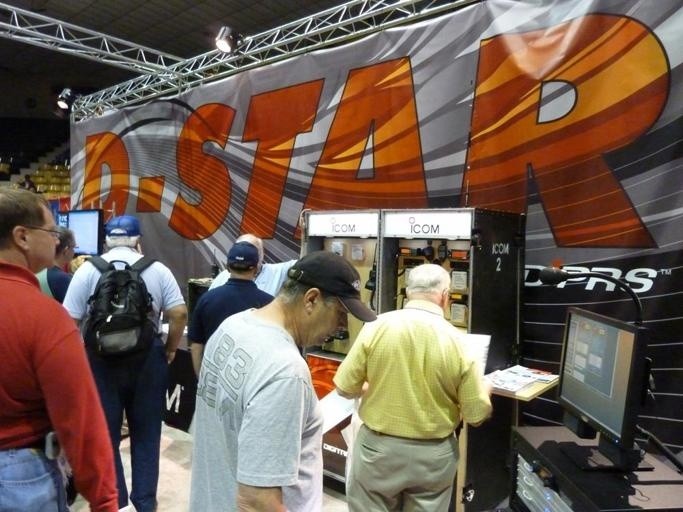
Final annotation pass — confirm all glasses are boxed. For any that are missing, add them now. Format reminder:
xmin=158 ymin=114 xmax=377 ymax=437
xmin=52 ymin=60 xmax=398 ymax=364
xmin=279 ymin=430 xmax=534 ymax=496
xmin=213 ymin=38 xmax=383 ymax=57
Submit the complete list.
xmin=22 ymin=225 xmax=64 ymax=240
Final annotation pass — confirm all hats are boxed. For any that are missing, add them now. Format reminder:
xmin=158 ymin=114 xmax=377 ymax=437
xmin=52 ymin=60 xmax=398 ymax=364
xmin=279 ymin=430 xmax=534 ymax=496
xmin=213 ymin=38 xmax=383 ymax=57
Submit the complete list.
xmin=287 ymin=250 xmax=377 ymax=321
xmin=104 ymin=216 xmax=141 ymax=237
xmin=228 ymin=242 xmax=258 ymax=270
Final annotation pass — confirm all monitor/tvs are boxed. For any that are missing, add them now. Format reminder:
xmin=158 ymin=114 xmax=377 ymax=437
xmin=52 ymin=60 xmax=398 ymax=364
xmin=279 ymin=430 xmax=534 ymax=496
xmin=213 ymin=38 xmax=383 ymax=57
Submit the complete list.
xmin=555 ymin=304 xmax=656 ymax=471
xmin=66 ymin=209 xmax=103 ymax=256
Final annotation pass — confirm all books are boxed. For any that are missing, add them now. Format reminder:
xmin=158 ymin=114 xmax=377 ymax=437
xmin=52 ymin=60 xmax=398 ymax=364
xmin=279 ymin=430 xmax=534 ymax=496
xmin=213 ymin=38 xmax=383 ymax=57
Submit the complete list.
xmin=485 ymin=363 xmax=559 ymax=402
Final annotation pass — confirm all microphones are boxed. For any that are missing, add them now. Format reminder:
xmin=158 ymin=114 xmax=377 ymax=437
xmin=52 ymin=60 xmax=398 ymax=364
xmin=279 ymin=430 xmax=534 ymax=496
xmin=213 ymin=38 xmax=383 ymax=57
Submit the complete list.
xmin=539 ymin=267 xmax=656 ymax=403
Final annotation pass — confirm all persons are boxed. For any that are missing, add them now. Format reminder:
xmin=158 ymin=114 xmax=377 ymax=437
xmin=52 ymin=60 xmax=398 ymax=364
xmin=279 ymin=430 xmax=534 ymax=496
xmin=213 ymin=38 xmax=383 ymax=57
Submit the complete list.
xmin=187 ymin=241 xmax=276 ymax=382
xmin=185 ymin=251 xmax=378 ymax=512
xmin=1 ymin=184 xmax=118 ymax=511
xmin=36 ymin=225 xmax=76 ymax=306
xmin=331 ymin=263 xmax=493 ymax=512
xmin=60 ymin=214 xmax=189 ymax=512
xmin=206 ymin=208 xmax=311 ymax=298
xmin=20 ymin=175 xmax=36 ymax=192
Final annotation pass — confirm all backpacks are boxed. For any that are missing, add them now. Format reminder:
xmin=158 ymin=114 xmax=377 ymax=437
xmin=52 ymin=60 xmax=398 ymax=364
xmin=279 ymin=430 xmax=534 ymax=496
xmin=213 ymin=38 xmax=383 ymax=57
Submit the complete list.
xmin=79 ymin=255 xmax=157 ymax=358
